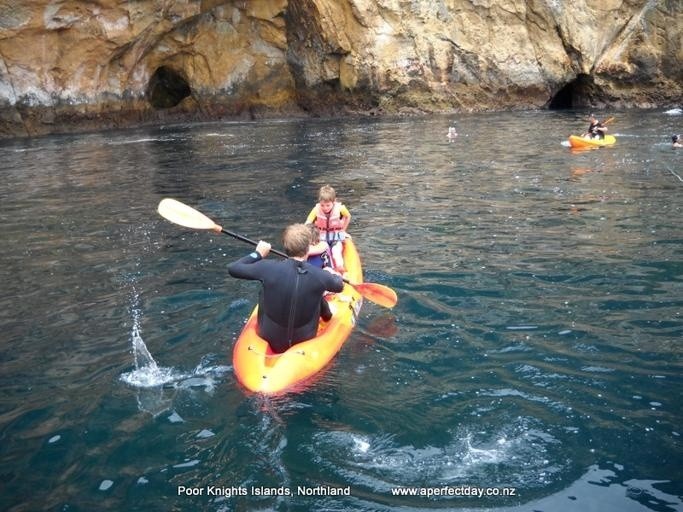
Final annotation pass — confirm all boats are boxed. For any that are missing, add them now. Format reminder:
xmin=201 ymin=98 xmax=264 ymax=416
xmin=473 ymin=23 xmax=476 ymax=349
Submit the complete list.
xmin=231 ymin=229 xmax=363 ymax=396
xmin=567 ymin=134 xmax=617 ymax=148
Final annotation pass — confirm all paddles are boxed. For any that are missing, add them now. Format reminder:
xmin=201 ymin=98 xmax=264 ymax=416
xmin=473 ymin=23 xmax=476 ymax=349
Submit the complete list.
xmin=581 ymin=116 xmax=614 ymax=136
xmin=157 ymin=198 xmax=398 ymax=308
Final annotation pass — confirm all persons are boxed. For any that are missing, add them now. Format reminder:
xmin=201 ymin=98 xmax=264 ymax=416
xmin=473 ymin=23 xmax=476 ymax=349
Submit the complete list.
xmin=226 ymin=223 xmax=343 ymax=353
xmin=671 ymin=134 xmax=683 ymax=148
xmin=304 ymin=185 xmax=350 ymax=272
xmin=585 ymin=113 xmax=607 ymax=140
